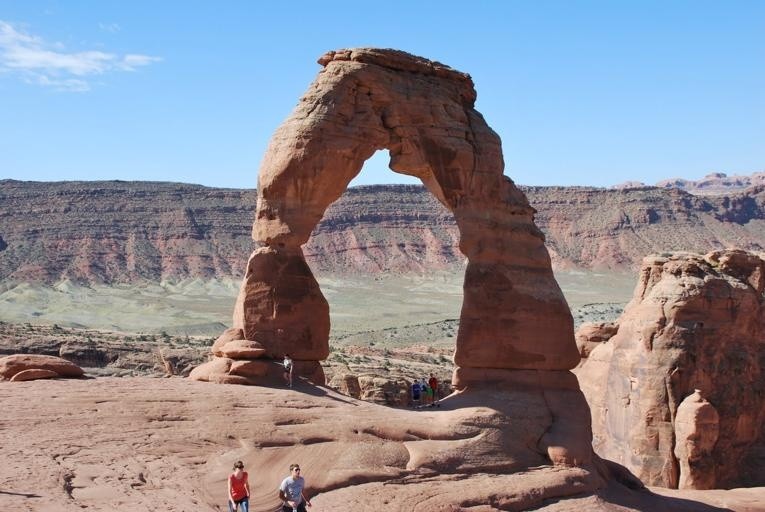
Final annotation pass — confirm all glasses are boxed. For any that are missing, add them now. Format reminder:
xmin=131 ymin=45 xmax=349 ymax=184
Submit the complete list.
xmin=236 ymin=466 xmax=244 ymax=469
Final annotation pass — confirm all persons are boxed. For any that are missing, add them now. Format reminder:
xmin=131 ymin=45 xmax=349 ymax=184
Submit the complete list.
xmin=420 ymin=378 xmax=428 ymax=405
xmin=282 ymin=353 xmax=294 ymax=387
xmin=279 ymin=464 xmax=313 ymax=512
xmin=426 ymin=383 xmax=432 ymax=404
xmin=411 ymin=379 xmax=420 ymax=409
xmin=429 ymin=373 xmax=440 ymax=407
xmin=228 ymin=460 xmax=250 ymax=511
